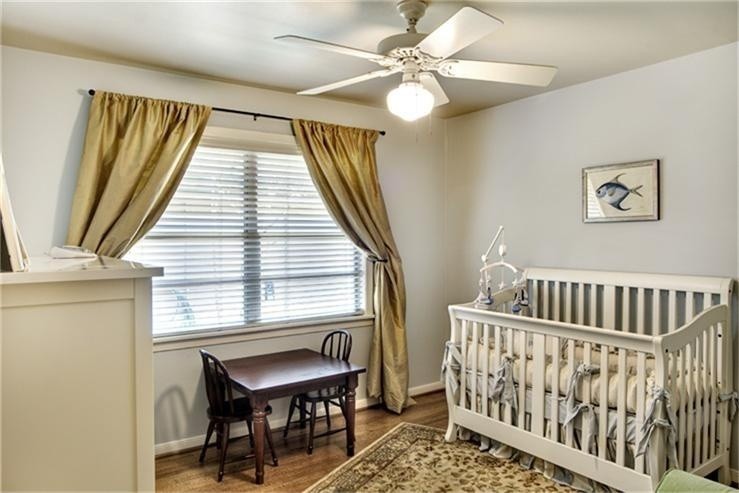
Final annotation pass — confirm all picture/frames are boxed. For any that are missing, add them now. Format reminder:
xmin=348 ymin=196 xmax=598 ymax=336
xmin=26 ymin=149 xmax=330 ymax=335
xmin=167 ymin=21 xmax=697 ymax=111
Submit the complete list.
xmin=582 ymin=157 xmax=661 ymax=225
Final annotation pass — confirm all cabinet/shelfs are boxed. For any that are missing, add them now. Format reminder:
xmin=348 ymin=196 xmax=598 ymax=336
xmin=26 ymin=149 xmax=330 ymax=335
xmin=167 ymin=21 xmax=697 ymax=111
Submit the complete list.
xmin=1 ymin=266 xmax=164 ymax=493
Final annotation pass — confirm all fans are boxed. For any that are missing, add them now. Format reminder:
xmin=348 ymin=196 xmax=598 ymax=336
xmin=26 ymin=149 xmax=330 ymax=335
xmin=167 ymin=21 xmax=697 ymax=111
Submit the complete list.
xmin=275 ymin=0 xmax=559 ymax=98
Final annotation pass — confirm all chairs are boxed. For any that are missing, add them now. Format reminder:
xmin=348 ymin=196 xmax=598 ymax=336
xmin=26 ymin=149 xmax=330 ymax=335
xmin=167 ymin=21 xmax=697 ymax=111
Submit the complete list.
xmin=284 ymin=330 xmax=353 ymax=454
xmin=199 ymin=348 xmax=278 ymax=481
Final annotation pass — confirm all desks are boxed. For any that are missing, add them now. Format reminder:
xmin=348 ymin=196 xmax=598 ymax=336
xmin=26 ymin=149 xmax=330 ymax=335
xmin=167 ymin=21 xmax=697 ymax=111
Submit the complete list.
xmin=206 ymin=348 xmax=367 ymax=484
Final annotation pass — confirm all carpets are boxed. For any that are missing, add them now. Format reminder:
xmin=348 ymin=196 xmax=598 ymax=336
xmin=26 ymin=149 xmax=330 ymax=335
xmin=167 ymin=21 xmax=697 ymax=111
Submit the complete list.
xmin=303 ymin=423 xmax=589 ymax=493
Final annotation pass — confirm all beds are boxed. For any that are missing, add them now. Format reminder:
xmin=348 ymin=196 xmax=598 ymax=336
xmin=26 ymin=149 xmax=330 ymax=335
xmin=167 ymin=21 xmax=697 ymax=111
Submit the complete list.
xmin=443 ymin=265 xmax=736 ymax=493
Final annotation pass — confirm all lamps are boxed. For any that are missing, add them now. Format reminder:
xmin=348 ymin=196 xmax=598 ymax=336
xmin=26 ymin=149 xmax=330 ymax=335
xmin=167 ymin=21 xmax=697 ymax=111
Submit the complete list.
xmin=385 ymin=63 xmax=434 ymax=122
